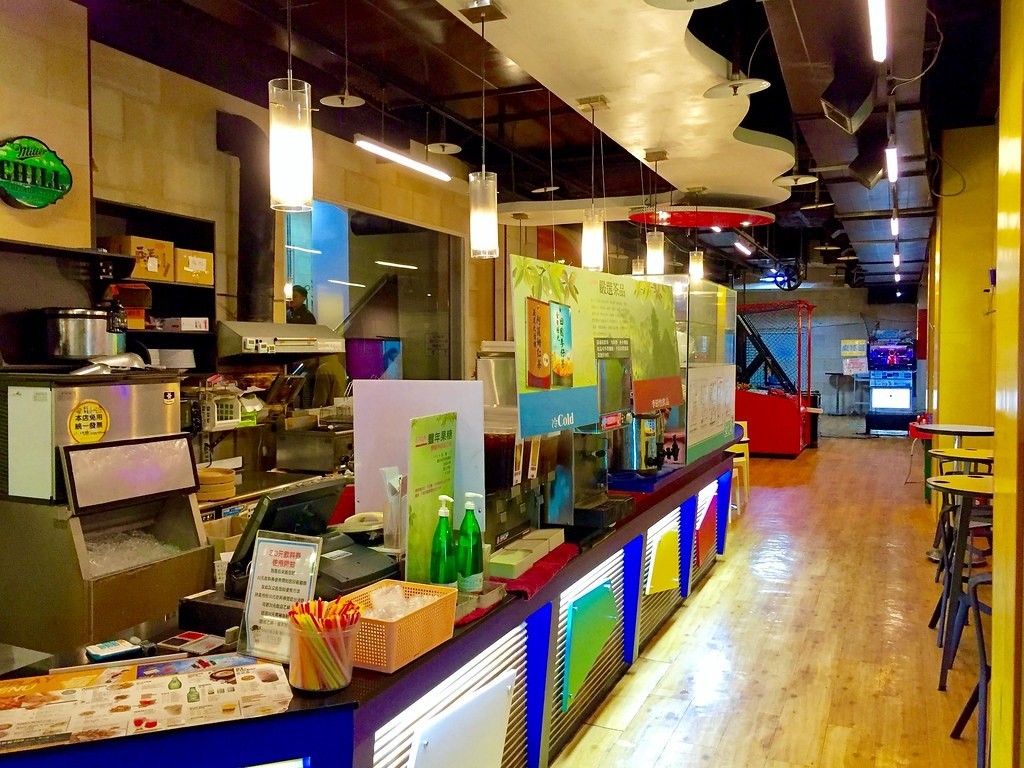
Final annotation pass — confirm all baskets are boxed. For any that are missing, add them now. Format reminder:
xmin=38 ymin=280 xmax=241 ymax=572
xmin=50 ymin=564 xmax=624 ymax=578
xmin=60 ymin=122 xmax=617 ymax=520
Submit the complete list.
xmin=325 ymin=578 xmax=459 ymax=675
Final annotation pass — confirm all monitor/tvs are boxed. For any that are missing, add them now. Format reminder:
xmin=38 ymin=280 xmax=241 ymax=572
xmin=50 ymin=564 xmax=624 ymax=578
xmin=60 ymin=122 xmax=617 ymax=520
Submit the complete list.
xmin=867 ymin=339 xmax=917 ymax=371
xmin=229 ymin=477 xmax=347 ymax=568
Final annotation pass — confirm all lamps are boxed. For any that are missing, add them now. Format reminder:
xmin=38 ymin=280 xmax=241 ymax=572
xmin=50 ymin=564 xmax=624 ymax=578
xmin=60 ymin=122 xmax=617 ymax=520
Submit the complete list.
xmin=686 ymin=187 xmax=707 ymax=280
xmin=269 ymin=0 xmax=314 ymax=211
xmin=989 ymin=268 xmax=996 ymax=286
xmin=459 ymin=0 xmax=507 ymax=258
xmin=645 ymin=150 xmax=665 ymax=274
xmin=577 ymin=94 xmax=609 ymax=271
xmin=353 ymin=84 xmax=452 ymax=181
xmin=860 ymin=0 xmax=903 ymax=281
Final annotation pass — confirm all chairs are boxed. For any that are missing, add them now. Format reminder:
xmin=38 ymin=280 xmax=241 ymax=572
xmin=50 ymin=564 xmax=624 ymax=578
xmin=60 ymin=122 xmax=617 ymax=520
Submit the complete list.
xmin=927 ymin=470 xmax=996 ymax=768
xmin=725 ymin=420 xmax=750 ymax=504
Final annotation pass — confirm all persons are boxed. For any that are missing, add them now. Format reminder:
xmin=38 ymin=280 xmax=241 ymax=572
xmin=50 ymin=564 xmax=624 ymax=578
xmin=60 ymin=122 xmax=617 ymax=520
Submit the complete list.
xmin=286 ymin=285 xmax=316 ymax=325
xmin=385 ymin=348 xmax=398 ymax=379
xmin=312 ymin=355 xmax=347 ymax=407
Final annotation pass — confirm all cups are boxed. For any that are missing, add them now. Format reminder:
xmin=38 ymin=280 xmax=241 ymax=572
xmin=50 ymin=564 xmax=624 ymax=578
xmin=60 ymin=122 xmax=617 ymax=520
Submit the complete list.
xmin=287 ymin=619 xmax=362 ymax=692
xmin=222 ymin=704 xmax=236 ymax=716
xmin=165 ymin=704 xmax=183 ymax=716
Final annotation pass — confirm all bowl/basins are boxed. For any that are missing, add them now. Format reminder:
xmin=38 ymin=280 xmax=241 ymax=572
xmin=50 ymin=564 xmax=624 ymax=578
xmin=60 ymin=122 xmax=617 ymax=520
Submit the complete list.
xmin=140 ymin=698 xmax=155 ymax=706
xmin=44 ymin=302 xmax=127 ymax=365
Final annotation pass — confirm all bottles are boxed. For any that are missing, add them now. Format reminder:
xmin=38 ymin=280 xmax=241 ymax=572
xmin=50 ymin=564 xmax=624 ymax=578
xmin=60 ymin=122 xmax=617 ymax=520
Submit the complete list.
xmin=168 ymin=677 xmax=182 ymax=689
xmin=186 ymin=687 xmax=200 ymax=704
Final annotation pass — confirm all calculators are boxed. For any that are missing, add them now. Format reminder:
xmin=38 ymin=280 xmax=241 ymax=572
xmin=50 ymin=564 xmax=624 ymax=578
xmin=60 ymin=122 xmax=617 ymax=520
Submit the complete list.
xmin=85 ymin=639 xmax=141 ymax=663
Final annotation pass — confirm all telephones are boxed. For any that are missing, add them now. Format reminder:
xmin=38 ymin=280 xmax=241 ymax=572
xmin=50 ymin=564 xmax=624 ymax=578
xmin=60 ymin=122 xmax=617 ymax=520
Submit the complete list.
xmin=326 ymin=511 xmax=384 ymax=547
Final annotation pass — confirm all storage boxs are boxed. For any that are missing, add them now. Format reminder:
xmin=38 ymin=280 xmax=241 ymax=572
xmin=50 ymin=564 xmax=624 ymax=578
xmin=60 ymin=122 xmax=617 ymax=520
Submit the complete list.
xmin=202 ymin=515 xmax=251 ymax=563
xmin=97 ymin=234 xmax=214 ymax=330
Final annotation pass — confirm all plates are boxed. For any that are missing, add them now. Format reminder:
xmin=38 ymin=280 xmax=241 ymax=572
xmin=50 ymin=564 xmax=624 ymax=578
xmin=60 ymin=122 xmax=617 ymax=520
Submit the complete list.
xmin=196 ymin=468 xmax=237 ymax=501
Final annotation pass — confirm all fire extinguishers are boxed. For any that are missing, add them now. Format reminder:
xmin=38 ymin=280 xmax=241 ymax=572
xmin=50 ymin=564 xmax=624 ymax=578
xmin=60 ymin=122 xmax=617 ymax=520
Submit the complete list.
xmin=970 ymin=496 xmax=987 ymax=536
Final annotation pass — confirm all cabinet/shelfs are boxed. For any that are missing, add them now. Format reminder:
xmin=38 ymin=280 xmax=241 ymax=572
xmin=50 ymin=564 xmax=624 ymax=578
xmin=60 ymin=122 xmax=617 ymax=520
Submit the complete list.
xmin=100 ymin=275 xmax=215 ymax=335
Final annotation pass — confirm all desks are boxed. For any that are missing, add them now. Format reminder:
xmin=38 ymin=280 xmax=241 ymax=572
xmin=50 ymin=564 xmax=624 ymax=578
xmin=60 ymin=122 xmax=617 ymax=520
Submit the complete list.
xmin=916 ymin=425 xmax=994 ymax=689
xmin=905 ymin=422 xmax=933 ymax=484
xmin=825 ymin=372 xmax=847 ymax=416
